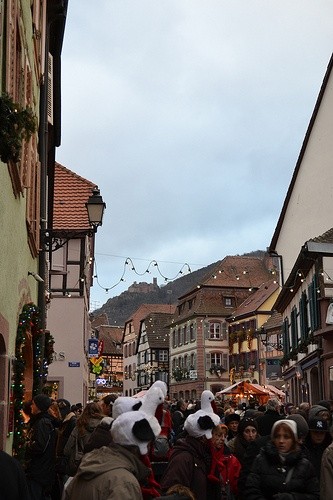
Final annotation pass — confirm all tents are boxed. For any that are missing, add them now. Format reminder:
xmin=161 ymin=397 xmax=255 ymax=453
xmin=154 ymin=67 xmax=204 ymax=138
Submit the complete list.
xmin=262 ymin=385 xmax=285 ymax=403
xmin=215 ymin=381 xmax=268 ymax=406
xmin=250 ymin=383 xmax=275 ymax=398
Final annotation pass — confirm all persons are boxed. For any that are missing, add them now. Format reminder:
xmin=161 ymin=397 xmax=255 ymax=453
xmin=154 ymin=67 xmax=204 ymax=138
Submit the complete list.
xmin=0 ymin=380 xmax=333 ymax=500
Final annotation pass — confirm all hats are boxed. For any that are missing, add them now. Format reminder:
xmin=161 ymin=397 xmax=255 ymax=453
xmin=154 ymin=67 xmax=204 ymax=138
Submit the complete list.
xmin=286 ymin=414 xmax=307 ymax=435
xmin=183 ymin=390 xmax=220 ymax=439
xmin=110 ymin=388 xmax=165 ymax=455
xmin=215 ymin=420 xmax=228 ymax=431
xmin=271 ymin=419 xmax=298 ymax=444
xmin=33 ymin=395 xmax=51 ymax=412
xmin=224 ymin=414 xmax=240 ymax=424
xmin=112 ymin=380 xmax=167 ymax=421
xmin=238 ymin=418 xmax=257 ymax=434
xmin=309 ymin=416 xmax=328 ymax=432
